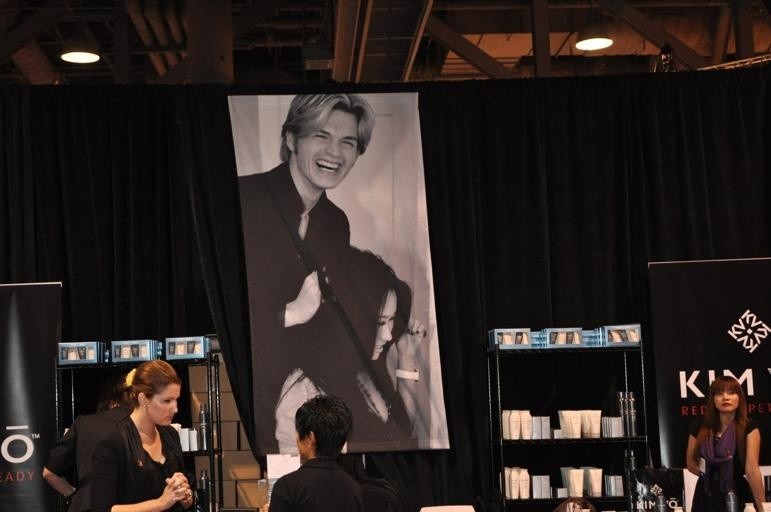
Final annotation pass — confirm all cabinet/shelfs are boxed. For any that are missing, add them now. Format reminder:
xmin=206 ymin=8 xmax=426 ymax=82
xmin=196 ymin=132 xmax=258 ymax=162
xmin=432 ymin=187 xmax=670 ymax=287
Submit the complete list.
xmin=488 ymin=340 xmax=652 ymax=511
xmin=50 ymin=352 xmax=224 ymax=510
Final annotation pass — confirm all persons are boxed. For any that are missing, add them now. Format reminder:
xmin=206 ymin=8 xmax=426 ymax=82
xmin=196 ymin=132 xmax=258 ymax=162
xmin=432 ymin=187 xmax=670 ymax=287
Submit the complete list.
xmin=41 ymin=369 xmax=136 ymax=511
xmin=237 ymin=92 xmax=376 ymax=454
xmin=71 ymin=361 xmax=197 ymax=512
xmin=686 ymin=376 xmax=766 ymax=512
xmin=263 ymin=394 xmax=365 ymax=512
xmin=268 ymin=246 xmax=428 ymax=453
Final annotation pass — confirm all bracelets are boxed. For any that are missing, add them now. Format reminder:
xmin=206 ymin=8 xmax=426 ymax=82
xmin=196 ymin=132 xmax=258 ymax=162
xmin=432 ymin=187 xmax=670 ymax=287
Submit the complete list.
xmin=394 ymin=368 xmax=420 ymax=383
xmin=64 ymin=487 xmax=76 ymax=499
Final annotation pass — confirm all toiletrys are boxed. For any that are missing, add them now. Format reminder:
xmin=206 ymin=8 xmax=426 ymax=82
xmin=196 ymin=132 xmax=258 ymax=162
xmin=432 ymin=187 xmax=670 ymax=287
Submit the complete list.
xmin=499 ymin=466 xmax=530 ymax=499
xmin=560 ymin=466 xmax=603 ymax=498
xmin=558 ymin=409 xmax=601 ymax=439
xmin=502 ymin=410 xmax=532 ymax=440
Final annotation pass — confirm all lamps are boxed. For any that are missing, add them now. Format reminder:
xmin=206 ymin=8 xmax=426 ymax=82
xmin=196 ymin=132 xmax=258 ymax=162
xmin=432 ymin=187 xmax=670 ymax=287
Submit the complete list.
xmin=60 ymin=27 xmax=102 ymax=65
xmin=573 ymin=2 xmax=616 ymax=52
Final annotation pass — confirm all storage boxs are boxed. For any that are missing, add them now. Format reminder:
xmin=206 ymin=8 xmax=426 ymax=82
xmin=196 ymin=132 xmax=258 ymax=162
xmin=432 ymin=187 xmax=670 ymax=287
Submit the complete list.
xmin=188 ymin=363 xmax=268 ymax=511
